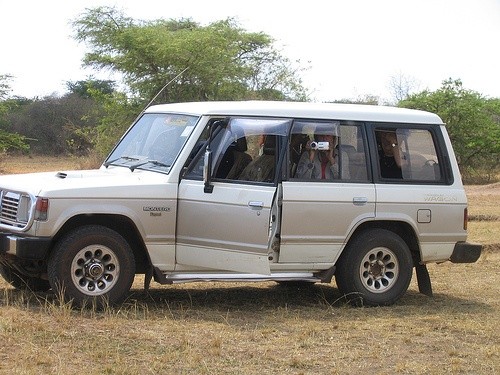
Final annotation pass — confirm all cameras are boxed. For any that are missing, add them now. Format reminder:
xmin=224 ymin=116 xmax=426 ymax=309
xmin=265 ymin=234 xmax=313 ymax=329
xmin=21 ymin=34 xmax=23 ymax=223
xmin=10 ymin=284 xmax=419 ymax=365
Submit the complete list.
xmin=310 ymin=141 xmax=329 ymax=151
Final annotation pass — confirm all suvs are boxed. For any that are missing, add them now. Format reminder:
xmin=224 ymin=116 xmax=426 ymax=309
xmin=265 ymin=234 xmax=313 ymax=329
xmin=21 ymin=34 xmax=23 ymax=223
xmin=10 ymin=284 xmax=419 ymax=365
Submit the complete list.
xmin=0 ymin=98 xmax=484 ymax=311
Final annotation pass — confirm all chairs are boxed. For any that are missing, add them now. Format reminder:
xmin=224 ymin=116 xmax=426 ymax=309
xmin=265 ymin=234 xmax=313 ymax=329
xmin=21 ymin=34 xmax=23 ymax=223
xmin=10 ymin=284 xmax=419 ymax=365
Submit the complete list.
xmin=334 ymin=143 xmax=365 ymax=181
xmin=238 ymin=125 xmax=278 ymax=181
xmin=399 ymin=152 xmax=434 ymax=182
xmin=287 ymin=129 xmax=312 ymax=178
xmin=192 ymin=123 xmax=253 ymax=182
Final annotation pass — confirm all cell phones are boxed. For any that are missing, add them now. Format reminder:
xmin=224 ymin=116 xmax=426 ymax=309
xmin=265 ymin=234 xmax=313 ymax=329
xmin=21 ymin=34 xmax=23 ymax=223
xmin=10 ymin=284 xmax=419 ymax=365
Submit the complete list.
xmin=392 ymin=144 xmax=404 ymax=166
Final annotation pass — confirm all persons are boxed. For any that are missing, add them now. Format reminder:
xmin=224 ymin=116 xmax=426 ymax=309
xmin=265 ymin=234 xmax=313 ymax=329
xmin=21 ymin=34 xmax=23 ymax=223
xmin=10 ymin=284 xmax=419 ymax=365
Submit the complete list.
xmin=293 ymin=124 xmax=352 ymax=181
xmin=354 ymin=124 xmax=414 ymax=182
xmin=254 ymin=122 xmax=275 ymax=153
